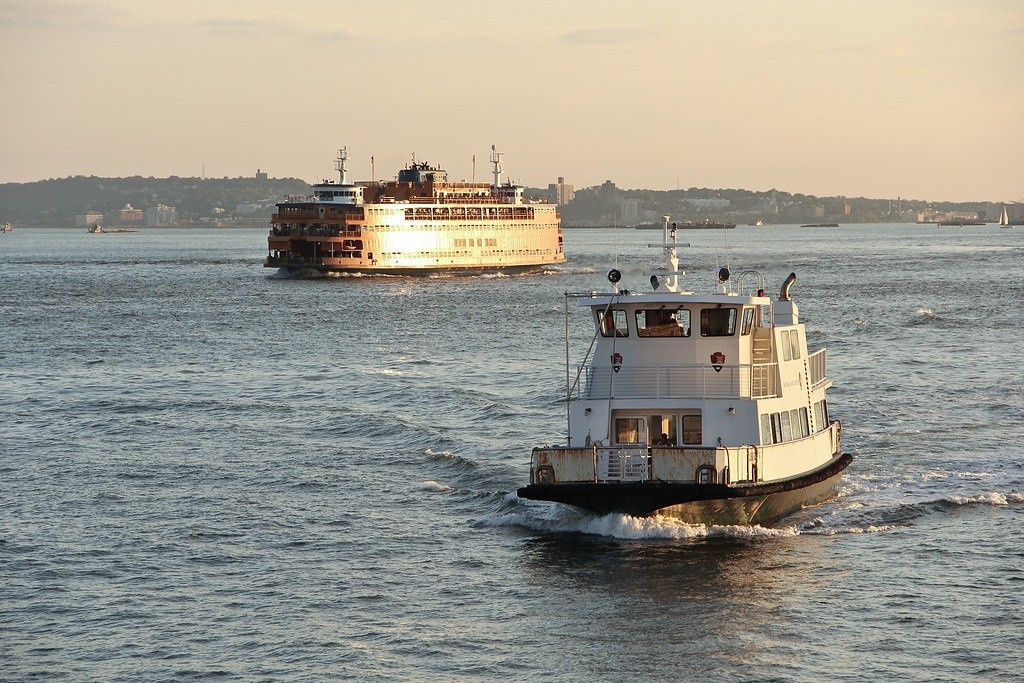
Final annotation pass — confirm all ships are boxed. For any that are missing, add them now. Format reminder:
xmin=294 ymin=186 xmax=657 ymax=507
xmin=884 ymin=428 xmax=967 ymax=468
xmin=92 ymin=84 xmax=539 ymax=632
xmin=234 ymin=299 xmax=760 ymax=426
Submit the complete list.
xmin=263 ymin=144 xmax=569 ymax=276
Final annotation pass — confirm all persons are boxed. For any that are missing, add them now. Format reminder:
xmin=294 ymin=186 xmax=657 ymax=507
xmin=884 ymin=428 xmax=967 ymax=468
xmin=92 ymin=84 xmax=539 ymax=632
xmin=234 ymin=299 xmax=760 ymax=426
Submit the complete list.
xmin=659 ymin=311 xmax=677 ymax=325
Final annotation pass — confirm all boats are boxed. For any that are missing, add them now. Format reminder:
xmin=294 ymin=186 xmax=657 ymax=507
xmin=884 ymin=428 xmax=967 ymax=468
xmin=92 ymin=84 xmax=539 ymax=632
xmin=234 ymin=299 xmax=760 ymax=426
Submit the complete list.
xmin=87 ymin=223 xmax=104 ymax=233
xmin=0 ymin=222 xmax=13 ymax=232
xmin=514 ymin=213 xmax=856 ymax=531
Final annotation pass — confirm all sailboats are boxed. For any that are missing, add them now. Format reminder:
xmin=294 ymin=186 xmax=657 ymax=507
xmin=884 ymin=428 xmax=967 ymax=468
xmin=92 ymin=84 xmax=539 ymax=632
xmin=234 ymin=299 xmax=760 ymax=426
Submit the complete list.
xmin=999 ymin=207 xmax=1015 ymax=229
